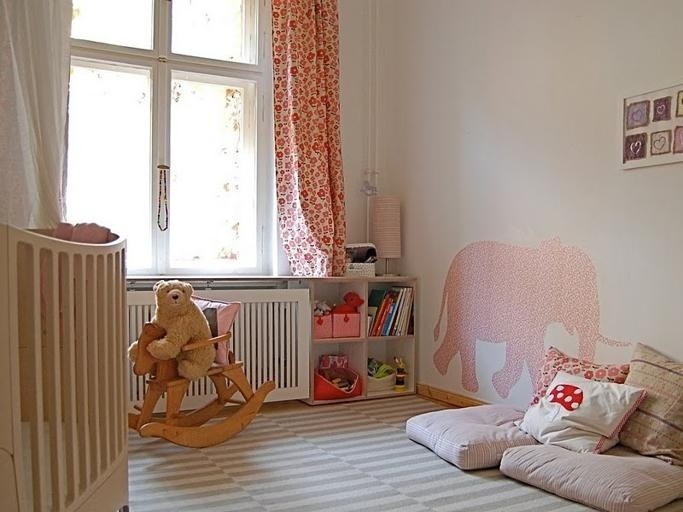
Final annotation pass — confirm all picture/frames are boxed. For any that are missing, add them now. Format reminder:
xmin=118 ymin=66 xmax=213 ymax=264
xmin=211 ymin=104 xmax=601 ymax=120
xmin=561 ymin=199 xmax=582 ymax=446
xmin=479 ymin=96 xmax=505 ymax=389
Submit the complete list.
xmin=618 ymin=77 xmax=683 ymax=171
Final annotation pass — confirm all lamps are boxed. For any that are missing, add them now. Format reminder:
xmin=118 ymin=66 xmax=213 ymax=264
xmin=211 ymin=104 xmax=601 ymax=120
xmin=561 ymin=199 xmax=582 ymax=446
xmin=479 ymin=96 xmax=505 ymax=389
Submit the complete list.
xmin=371 ymin=197 xmax=402 ymax=276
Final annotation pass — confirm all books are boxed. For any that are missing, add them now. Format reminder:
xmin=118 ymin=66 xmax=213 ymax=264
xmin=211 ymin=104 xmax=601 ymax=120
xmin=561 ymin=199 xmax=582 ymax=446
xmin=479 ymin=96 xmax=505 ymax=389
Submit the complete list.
xmin=368 ymin=285 xmax=413 ymax=335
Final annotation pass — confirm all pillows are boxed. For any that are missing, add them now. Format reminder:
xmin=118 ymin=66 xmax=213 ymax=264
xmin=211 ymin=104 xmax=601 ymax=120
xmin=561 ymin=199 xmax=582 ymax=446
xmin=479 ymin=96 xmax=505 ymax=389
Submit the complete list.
xmin=512 ymin=342 xmax=683 ymax=467
xmin=191 ymin=295 xmax=242 ymax=368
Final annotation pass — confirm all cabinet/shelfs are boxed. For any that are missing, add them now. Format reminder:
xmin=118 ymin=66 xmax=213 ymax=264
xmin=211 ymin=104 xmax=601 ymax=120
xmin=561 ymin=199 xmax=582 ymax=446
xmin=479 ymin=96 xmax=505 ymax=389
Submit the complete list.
xmin=287 ymin=279 xmax=417 ymax=407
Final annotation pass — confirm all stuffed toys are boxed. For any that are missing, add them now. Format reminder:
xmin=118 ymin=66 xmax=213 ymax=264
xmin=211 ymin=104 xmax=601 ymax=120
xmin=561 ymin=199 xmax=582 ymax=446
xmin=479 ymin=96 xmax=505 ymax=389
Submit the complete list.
xmin=128 ymin=278 xmax=216 ymax=380
